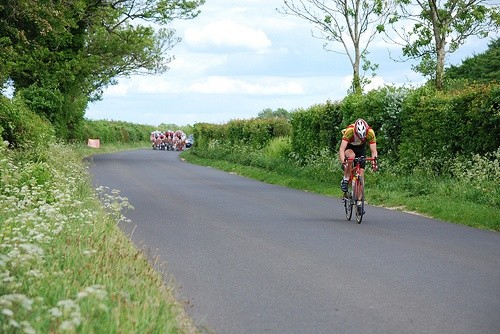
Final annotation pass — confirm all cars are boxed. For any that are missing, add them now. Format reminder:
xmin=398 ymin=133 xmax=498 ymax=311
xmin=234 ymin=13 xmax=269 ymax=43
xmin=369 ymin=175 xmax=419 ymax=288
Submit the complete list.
xmin=186 ymin=135 xmax=194 ymax=147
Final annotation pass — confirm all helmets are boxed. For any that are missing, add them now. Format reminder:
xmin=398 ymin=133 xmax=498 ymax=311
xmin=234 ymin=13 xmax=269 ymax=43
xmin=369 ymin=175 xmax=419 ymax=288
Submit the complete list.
xmin=354 ymin=118 xmax=369 ymax=142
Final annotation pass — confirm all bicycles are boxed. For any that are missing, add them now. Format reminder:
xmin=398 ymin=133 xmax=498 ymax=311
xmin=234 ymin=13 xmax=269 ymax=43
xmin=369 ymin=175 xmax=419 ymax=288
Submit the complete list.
xmin=343 ymin=156 xmax=378 ymax=223
xmin=152 ymin=138 xmax=183 ymax=151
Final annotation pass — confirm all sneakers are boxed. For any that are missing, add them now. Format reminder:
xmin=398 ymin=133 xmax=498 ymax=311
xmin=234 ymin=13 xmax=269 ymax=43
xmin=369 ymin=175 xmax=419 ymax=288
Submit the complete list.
xmin=341 ymin=180 xmax=349 ymax=192
xmin=356 ymin=205 xmax=365 ymax=215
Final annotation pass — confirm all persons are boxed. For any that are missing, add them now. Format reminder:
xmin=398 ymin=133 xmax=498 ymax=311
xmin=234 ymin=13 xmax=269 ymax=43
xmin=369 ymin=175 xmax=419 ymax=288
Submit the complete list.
xmin=149 ymin=129 xmax=186 ymax=151
xmin=339 ymin=117 xmax=377 ymax=215
